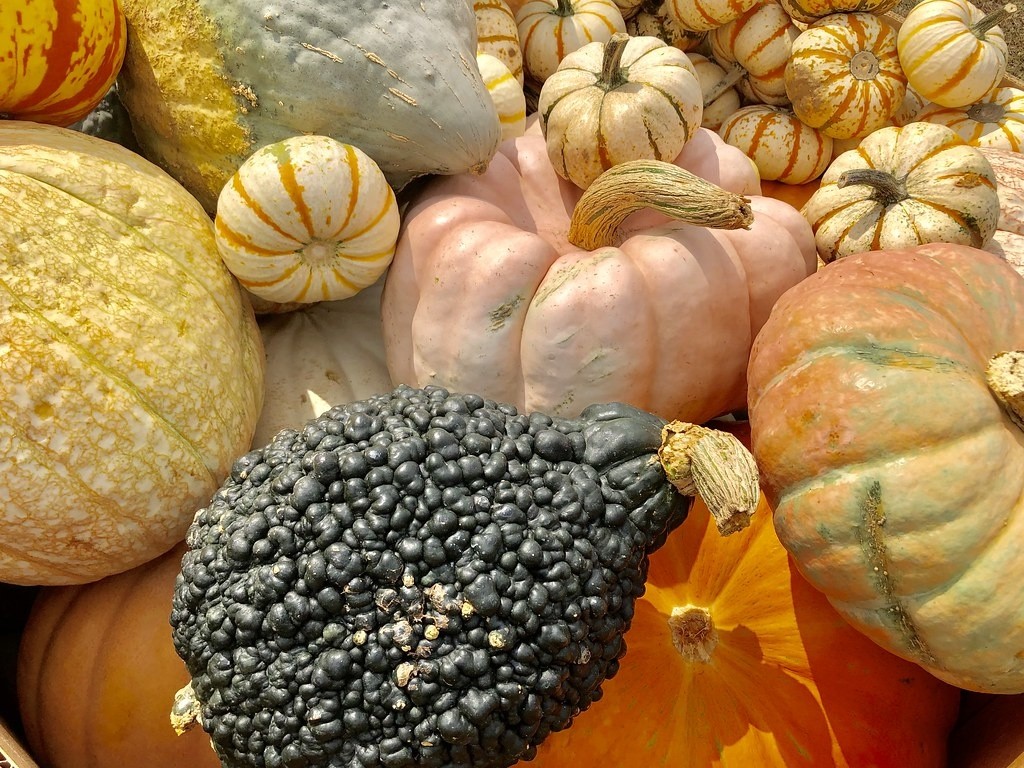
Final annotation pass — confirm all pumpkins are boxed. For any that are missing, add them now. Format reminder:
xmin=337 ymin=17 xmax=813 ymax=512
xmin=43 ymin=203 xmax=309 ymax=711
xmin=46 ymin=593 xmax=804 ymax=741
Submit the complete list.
xmin=0 ymin=0 xmax=1024 ymax=768
